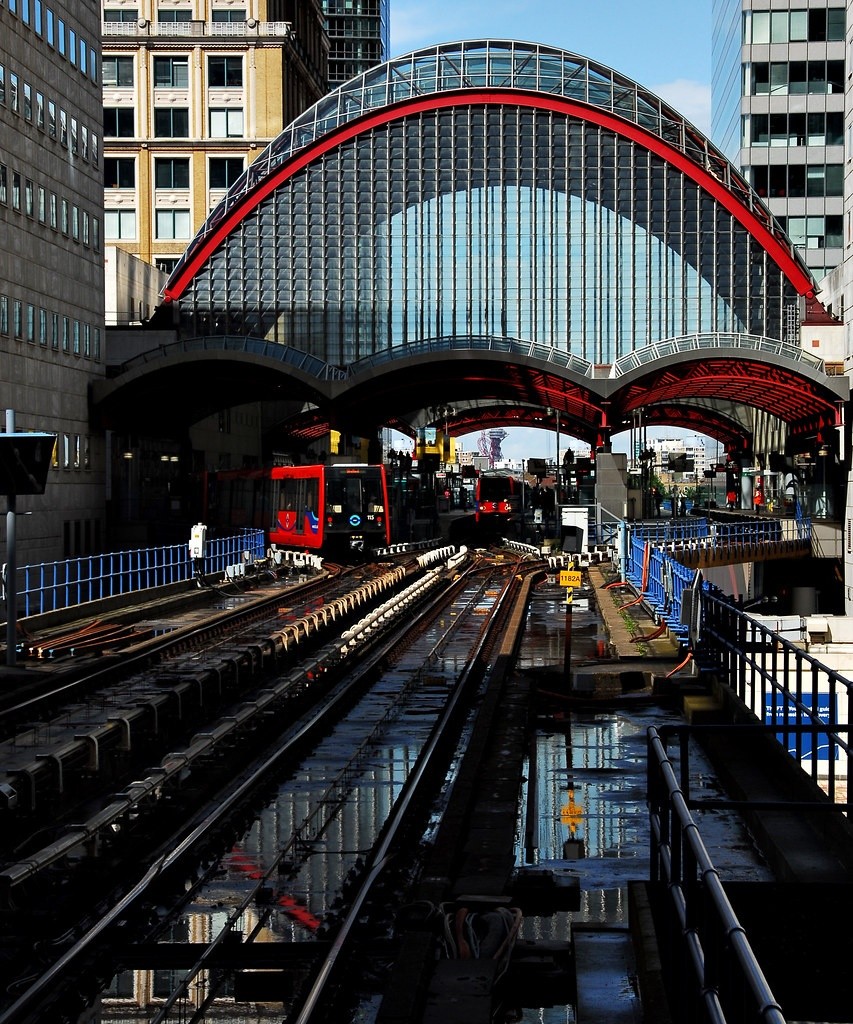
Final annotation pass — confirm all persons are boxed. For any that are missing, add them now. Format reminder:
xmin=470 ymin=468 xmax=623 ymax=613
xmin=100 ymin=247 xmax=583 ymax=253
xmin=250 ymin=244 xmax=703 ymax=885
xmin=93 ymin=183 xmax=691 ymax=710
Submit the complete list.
xmin=444 ymin=488 xmax=451 ymax=514
xmin=753 ymin=487 xmax=764 ymax=516
xmin=530 ymin=485 xmax=554 ymax=523
xmin=461 ymin=487 xmax=469 ymax=511
xmin=653 ymin=486 xmax=664 ymax=519
xmin=728 ymin=490 xmax=736 ymax=511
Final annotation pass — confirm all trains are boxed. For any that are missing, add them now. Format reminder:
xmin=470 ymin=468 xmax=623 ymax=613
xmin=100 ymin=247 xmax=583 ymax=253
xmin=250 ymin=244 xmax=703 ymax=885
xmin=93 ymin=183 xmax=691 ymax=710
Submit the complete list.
xmin=207 ymin=464 xmax=389 ymax=566
xmin=474 ymin=475 xmax=528 ymax=524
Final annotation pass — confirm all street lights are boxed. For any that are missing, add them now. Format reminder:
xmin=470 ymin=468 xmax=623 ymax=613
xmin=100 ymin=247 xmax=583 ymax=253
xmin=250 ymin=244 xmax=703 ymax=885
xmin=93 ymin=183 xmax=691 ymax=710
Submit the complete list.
xmin=697 ymin=484 xmax=700 ymax=504
xmin=0 ymin=410 xmax=57 ymax=667
xmin=546 ymin=407 xmax=560 ymax=521
